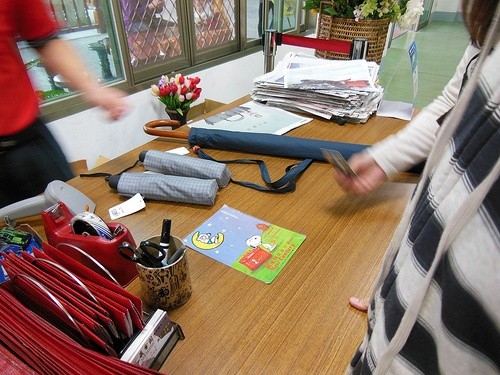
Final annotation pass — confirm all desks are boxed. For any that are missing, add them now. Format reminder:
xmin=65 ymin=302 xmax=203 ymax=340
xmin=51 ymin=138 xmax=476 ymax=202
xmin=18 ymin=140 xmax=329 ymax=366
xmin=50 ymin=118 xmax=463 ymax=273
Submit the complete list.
xmin=0 ymin=91 xmax=419 ymax=375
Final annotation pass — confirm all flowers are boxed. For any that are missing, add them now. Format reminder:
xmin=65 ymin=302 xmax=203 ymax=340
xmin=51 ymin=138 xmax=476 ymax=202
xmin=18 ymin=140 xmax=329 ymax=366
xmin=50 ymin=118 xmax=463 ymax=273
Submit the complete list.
xmin=300 ymin=0 xmax=425 ymax=35
xmin=149 ymin=70 xmax=203 ymax=117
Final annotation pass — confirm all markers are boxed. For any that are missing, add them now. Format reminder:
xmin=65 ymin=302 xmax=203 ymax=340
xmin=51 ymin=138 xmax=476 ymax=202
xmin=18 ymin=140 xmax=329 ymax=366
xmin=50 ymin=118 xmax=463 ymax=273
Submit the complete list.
xmin=158 ymin=219 xmax=171 ymax=266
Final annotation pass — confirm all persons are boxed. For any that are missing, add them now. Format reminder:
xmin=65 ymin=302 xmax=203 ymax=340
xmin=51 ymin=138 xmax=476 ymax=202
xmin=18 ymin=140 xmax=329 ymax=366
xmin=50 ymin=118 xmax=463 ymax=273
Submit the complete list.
xmin=333 ymin=0 xmax=500 ymax=375
xmin=193 ymin=0 xmax=218 ymax=33
xmin=120 ymin=0 xmax=175 ymax=33
xmin=0 ymin=0 xmax=126 ymax=210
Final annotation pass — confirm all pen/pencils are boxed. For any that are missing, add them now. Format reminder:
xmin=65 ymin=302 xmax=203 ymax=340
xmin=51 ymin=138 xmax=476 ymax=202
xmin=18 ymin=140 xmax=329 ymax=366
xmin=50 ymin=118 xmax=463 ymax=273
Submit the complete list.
xmin=168 ymin=245 xmax=185 ymax=265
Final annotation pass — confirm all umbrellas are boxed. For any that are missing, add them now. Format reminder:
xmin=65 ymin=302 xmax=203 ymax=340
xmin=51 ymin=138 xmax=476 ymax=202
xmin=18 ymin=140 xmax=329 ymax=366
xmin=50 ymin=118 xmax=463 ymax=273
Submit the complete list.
xmin=144 ymin=118 xmax=426 ymax=173
xmin=139 ymin=150 xmax=232 ymax=187
xmin=106 ymin=172 xmax=219 ymax=206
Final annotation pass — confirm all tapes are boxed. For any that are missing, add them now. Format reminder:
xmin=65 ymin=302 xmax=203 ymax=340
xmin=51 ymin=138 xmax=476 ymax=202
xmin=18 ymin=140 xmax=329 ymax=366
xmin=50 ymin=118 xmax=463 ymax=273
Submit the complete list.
xmin=70 ymin=211 xmax=112 ymax=240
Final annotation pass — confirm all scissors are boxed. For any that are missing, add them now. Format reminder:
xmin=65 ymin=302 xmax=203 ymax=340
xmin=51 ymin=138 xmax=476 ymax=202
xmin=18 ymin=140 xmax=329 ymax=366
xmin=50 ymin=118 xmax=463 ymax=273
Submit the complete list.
xmin=117 ymin=240 xmax=166 ymax=267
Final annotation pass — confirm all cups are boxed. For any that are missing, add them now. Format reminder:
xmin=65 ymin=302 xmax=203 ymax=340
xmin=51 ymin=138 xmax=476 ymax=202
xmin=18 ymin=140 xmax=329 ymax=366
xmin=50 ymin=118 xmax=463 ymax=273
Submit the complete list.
xmin=133 ymin=233 xmax=194 ymax=310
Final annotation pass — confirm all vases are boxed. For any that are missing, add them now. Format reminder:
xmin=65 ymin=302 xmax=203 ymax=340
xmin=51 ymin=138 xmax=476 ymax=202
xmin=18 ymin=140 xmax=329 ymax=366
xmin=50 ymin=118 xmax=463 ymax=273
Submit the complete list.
xmin=164 ymin=106 xmax=191 ymax=129
